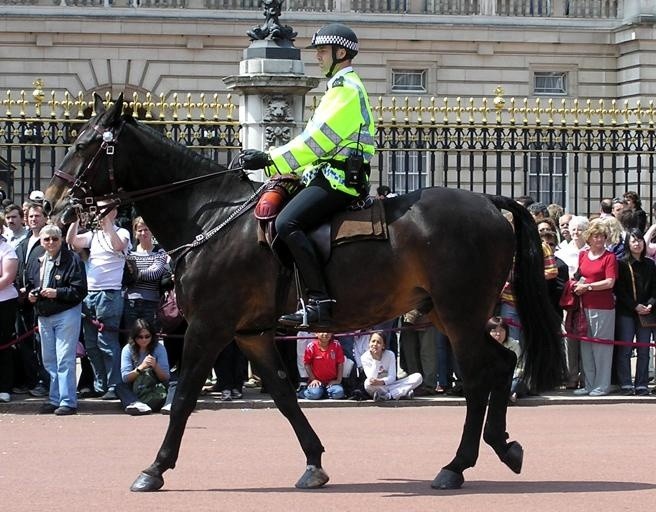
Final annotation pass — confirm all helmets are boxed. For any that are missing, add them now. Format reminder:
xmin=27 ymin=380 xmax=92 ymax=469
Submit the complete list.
xmin=306 ymin=24 xmax=359 ymax=54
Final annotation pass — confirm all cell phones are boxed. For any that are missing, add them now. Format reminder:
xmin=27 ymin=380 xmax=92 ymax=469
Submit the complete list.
xmin=577 ymin=277 xmax=586 ymax=285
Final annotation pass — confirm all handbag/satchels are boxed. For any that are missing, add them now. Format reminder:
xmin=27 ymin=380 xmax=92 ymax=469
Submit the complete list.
xmin=159 ymin=290 xmax=186 ymax=329
xmin=639 ymin=314 xmax=656 ymax=328
xmin=565 ymin=294 xmax=588 ymax=336
xmin=132 ymin=368 xmax=167 ymax=411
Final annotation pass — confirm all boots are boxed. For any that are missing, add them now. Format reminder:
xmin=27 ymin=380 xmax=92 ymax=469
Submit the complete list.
xmin=278 ymin=230 xmax=332 ymax=327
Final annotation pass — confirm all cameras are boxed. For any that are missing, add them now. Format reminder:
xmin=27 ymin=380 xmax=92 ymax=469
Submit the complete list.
xmin=31 ymin=286 xmax=43 ymax=297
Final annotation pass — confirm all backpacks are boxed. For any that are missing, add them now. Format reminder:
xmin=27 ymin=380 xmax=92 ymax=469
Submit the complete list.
xmin=122 ymin=260 xmax=138 ymax=288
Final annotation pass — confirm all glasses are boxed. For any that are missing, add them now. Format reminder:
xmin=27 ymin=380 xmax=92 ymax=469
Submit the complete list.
xmin=44 ymin=238 xmax=58 ymax=241
xmin=135 ymin=334 xmax=151 ymax=339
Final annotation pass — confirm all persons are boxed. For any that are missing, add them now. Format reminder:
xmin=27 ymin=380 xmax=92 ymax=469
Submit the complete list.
xmin=0 ymin=189 xmax=655 ymax=415
xmin=238 ymin=24 xmax=376 ymax=329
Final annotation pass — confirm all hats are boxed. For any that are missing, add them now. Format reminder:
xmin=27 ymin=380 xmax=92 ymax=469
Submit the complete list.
xmin=30 ymin=190 xmax=45 ymax=201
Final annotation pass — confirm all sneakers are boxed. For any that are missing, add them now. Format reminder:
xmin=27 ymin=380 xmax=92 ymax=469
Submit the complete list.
xmin=404 ymin=390 xmax=414 ymax=399
xmin=374 ymin=392 xmax=391 ymax=401
xmin=244 ymin=375 xmax=261 ymax=387
xmin=297 ymin=382 xmax=307 ymax=393
xmin=231 ymin=390 xmax=242 ymax=398
xmin=589 ymin=387 xmax=608 ymax=395
xmin=160 ymin=403 xmax=171 ymax=414
xmin=573 ymin=388 xmax=589 ymax=395
xmin=414 ymin=389 xmax=431 ymax=395
xmin=38 ymin=404 xmax=57 ymax=414
xmin=221 ymin=390 xmax=232 ymax=401
xmin=28 ymin=386 xmax=49 ymax=396
xmin=0 ymin=393 xmax=11 ymax=402
xmin=103 ymin=391 xmax=118 ymax=399
xmin=447 ymin=382 xmax=463 ymax=394
xmin=55 ymin=406 xmax=77 ymax=414
xmin=125 ymin=402 xmax=152 ymax=415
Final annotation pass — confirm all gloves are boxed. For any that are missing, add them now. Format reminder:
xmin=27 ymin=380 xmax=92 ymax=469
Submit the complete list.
xmin=241 ymin=150 xmax=267 ymax=170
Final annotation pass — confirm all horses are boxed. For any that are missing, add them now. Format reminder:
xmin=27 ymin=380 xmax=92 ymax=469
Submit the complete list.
xmin=42 ymin=91 xmax=567 ymax=495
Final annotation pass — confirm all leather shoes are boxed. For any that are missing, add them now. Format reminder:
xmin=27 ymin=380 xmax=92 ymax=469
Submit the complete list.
xmin=620 ymin=386 xmax=634 ymax=395
xmin=635 ymin=386 xmax=649 ymax=395
